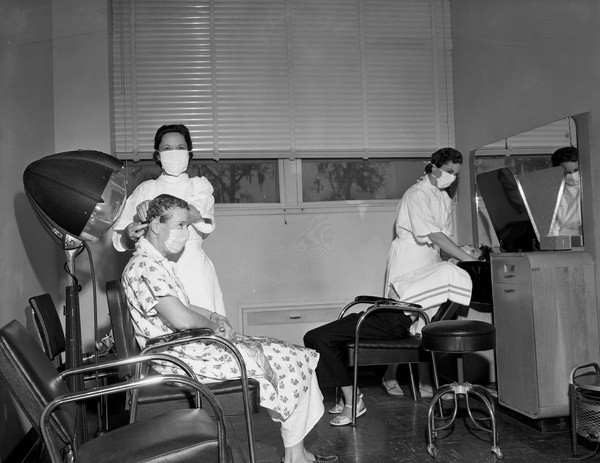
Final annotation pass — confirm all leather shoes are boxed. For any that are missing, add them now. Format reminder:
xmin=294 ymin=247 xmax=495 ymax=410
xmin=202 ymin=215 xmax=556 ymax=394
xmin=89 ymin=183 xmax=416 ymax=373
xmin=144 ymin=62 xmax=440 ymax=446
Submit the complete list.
xmin=281 ymin=452 xmax=339 ymax=463
xmin=381 ymin=375 xmax=404 ymax=398
xmin=419 ymin=386 xmax=455 ymax=400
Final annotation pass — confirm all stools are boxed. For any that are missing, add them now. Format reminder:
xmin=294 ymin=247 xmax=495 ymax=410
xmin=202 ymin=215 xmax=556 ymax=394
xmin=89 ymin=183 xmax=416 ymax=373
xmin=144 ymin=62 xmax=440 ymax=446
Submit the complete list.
xmin=421 ymin=319 xmax=504 ymax=461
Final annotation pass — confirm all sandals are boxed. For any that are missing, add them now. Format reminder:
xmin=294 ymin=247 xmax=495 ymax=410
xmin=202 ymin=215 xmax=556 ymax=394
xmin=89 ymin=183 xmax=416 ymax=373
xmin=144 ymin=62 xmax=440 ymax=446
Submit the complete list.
xmin=328 ymin=392 xmax=364 ymax=414
xmin=330 ymin=398 xmax=367 ymax=426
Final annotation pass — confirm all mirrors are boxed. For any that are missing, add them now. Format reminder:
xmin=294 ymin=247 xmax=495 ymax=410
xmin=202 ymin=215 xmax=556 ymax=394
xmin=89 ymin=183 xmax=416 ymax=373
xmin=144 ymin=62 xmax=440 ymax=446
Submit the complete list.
xmin=473 ymin=115 xmax=585 ymax=249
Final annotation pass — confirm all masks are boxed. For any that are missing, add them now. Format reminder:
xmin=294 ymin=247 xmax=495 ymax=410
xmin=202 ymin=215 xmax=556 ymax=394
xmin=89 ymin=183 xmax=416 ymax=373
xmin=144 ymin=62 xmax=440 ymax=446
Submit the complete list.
xmin=156 ymin=222 xmax=190 ymax=255
xmin=423 ymin=160 xmax=457 ymax=189
xmin=564 ymin=171 xmax=580 ymax=186
xmin=153 ymin=148 xmax=192 ymax=176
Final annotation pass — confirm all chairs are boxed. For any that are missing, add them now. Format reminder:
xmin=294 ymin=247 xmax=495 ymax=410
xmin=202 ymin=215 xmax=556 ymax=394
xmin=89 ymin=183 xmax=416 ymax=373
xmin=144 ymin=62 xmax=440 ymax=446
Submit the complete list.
xmin=107 ymin=280 xmax=261 ymax=462
xmin=1 ymin=319 xmax=232 ymax=462
xmin=334 ymin=267 xmax=477 ymax=425
xmin=28 ymin=293 xmax=121 ymax=439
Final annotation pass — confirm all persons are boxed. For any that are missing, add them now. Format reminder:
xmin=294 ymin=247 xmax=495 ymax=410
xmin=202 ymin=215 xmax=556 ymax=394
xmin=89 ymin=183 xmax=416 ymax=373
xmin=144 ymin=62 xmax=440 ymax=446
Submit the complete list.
xmin=549 ymin=146 xmax=582 ymax=236
xmin=304 ymin=244 xmax=494 ymax=426
xmin=381 ymin=148 xmax=486 ymax=400
xmin=120 ymin=194 xmax=339 ymax=462
xmin=112 ymin=124 xmax=225 ymax=318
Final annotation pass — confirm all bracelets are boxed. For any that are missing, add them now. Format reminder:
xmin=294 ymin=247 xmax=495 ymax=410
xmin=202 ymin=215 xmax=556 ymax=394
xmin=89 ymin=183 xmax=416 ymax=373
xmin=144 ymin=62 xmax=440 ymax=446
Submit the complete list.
xmin=209 ymin=311 xmax=217 ymax=319
